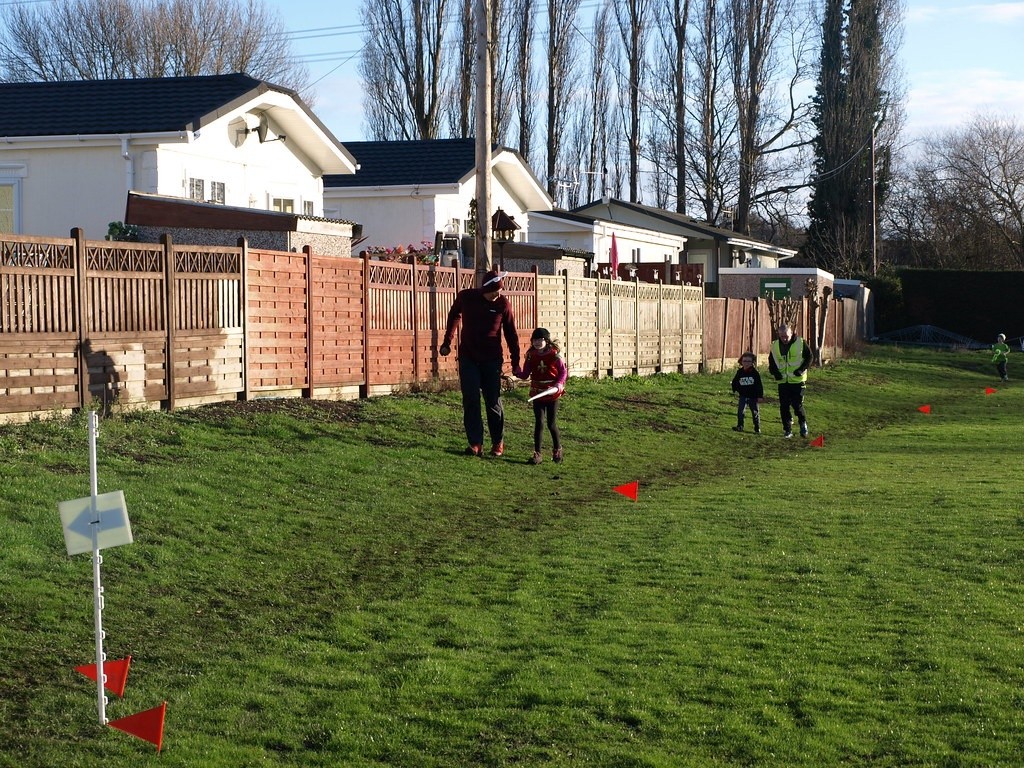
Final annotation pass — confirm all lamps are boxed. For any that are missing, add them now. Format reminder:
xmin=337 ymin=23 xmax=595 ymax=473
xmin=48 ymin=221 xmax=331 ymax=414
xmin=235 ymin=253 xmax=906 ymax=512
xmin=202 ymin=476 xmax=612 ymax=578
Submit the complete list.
xmin=264 ymin=135 xmax=287 ymax=144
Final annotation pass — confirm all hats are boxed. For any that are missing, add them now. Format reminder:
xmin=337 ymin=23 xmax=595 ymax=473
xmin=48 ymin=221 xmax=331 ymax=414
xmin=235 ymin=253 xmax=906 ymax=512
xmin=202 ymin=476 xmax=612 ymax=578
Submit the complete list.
xmin=532 ymin=328 xmax=550 ymax=339
xmin=482 ymin=270 xmax=503 ymax=293
xmin=999 ymin=334 xmax=1006 ymax=341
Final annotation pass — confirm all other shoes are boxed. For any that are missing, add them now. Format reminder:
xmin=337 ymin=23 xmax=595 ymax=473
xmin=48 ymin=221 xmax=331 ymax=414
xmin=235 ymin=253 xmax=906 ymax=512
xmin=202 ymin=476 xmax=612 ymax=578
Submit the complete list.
xmin=755 ymin=428 xmax=761 ymax=433
xmin=732 ymin=425 xmax=744 ymax=433
xmin=1004 ymin=374 xmax=1008 ymax=380
xmin=784 ymin=431 xmax=793 ymax=438
xmin=532 ymin=451 xmax=542 ymax=464
xmin=553 ymin=445 xmax=562 ymax=462
xmin=800 ymin=423 xmax=808 ymax=437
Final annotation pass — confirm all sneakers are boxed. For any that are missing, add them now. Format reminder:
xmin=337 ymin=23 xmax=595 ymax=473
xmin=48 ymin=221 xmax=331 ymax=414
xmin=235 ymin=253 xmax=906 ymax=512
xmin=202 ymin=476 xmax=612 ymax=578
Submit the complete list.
xmin=491 ymin=438 xmax=504 ymax=455
xmin=466 ymin=443 xmax=483 ymax=455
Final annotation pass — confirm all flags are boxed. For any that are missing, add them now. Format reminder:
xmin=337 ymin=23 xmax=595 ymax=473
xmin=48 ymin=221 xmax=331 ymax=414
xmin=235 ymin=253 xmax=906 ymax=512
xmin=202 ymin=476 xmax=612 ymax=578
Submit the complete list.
xmin=611 ymin=233 xmax=618 ymax=279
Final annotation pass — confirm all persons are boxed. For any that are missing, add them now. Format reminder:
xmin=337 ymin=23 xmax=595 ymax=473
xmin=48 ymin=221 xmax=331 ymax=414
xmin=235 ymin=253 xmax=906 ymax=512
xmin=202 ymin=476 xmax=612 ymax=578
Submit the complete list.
xmin=514 ymin=328 xmax=567 ymax=464
xmin=731 ymin=352 xmax=764 ymax=434
xmin=440 ymin=271 xmax=520 ymax=456
xmin=994 ymin=334 xmax=1010 ymax=383
xmin=767 ymin=324 xmax=813 ymax=438
